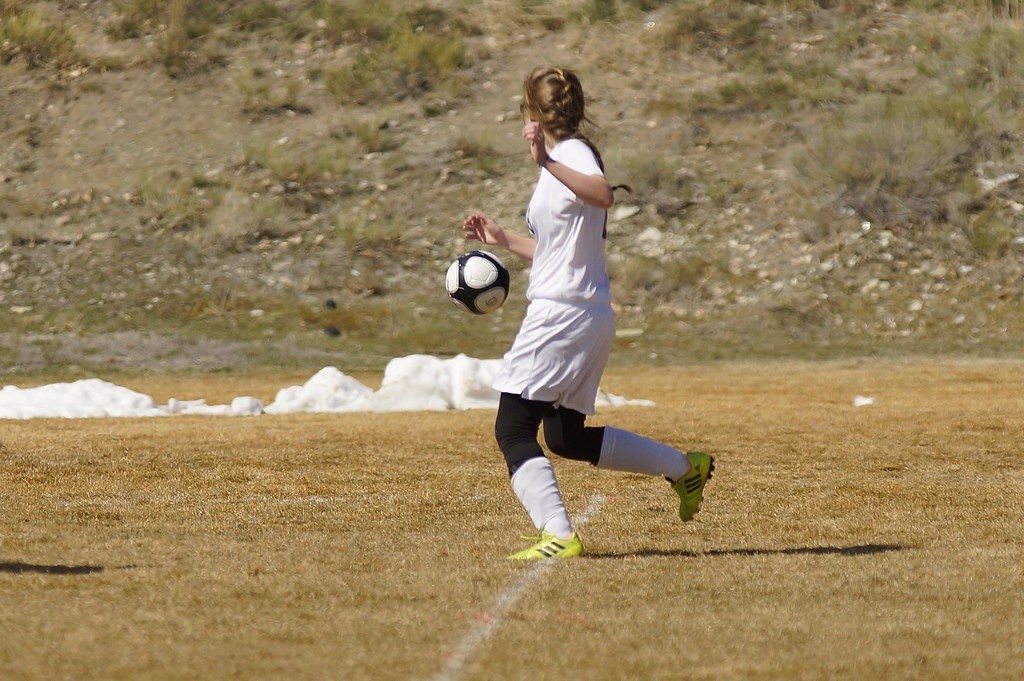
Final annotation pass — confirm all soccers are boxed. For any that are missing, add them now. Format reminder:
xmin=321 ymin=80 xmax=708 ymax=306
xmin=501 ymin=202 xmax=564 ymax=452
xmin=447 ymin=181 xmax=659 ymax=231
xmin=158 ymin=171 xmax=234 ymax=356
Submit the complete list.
xmin=444 ymin=250 xmax=511 ymax=315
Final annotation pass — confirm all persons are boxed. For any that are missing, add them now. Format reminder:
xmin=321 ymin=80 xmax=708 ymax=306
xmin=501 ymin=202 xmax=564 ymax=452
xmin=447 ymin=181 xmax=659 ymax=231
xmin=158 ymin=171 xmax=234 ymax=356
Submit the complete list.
xmin=463 ymin=66 xmax=715 ymax=560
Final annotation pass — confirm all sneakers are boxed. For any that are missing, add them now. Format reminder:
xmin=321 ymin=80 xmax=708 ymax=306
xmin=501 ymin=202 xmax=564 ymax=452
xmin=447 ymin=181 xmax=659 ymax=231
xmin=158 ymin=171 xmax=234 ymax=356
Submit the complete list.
xmin=506 ymin=531 xmax=583 ymax=561
xmin=664 ymin=451 xmax=715 ymax=522
